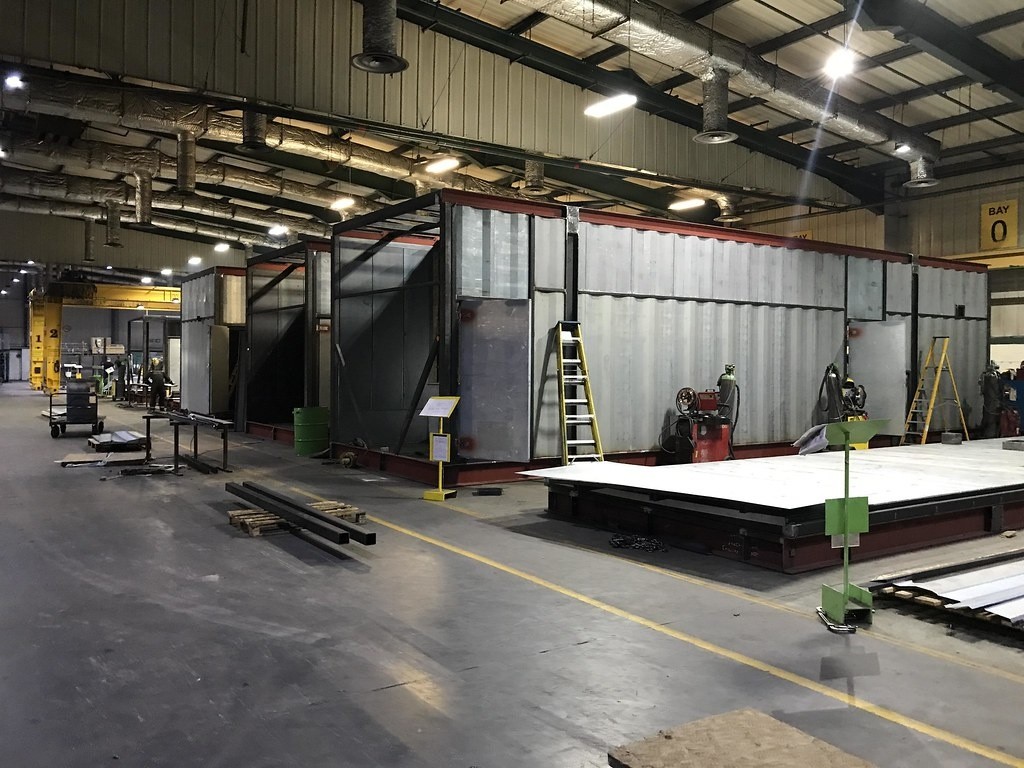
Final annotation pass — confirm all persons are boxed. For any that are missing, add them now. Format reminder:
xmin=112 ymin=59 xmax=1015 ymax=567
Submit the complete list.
xmin=102 ymin=357 xmax=114 ymax=378
xmin=143 ymin=357 xmax=174 ymax=414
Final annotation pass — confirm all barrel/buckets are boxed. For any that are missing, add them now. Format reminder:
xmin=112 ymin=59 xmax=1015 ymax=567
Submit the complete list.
xmin=292 ymin=407 xmax=329 ymax=457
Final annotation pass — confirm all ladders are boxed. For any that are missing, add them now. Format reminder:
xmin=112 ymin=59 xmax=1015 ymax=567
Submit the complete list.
xmin=899 ymin=335 xmax=970 ymax=445
xmin=555 ymin=321 xmax=605 ymax=466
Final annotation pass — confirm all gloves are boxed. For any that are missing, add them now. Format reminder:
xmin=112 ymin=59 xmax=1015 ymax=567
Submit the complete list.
xmin=169 ymin=381 xmax=174 ymax=385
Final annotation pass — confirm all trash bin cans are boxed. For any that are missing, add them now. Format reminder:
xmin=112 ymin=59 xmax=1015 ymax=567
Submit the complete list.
xmin=294 ymin=406 xmax=330 ymax=459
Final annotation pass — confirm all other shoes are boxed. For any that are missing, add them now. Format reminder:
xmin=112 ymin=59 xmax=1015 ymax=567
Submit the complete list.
xmin=147 ymin=410 xmax=153 ymax=414
xmin=159 ymin=411 xmax=165 ymax=414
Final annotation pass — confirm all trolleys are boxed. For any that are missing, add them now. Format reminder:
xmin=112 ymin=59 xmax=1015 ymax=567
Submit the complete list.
xmin=49 ymin=392 xmax=106 ymax=438
